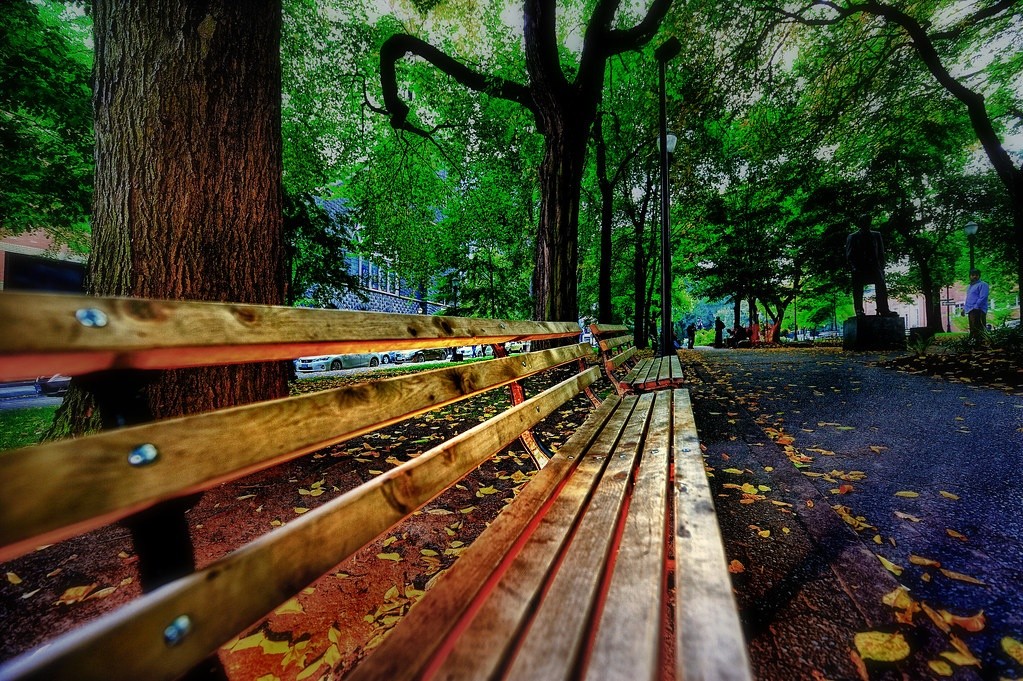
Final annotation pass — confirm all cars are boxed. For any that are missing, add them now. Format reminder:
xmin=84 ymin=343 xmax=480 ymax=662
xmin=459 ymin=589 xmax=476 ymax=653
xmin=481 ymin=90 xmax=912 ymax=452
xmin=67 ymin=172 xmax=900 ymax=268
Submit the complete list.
xmin=33 ymin=377 xmax=71 ymax=398
xmin=293 ymin=340 xmax=531 ymax=374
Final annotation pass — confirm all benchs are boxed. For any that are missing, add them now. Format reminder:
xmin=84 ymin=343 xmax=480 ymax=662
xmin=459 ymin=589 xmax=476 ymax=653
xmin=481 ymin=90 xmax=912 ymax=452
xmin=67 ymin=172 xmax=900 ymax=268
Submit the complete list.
xmin=1 ymin=289 xmax=755 ymax=681
xmin=590 ymin=322 xmax=685 ymax=398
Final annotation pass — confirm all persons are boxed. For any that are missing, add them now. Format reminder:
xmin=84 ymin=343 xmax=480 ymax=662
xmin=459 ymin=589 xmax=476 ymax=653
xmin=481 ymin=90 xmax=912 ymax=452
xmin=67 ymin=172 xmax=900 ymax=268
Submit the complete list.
xmin=961 ymin=268 xmax=990 ymax=339
xmin=687 ymin=322 xmax=696 ymax=349
xmin=715 ymin=316 xmax=724 ymax=348
xmin=671 ymin=322 xmax=681 ymax=347
xmin=724 ymin=329 xmax=735 ymax=347
xmin=846 ymin=213 xmax=891 ymax=316
xmin=732 ymin=324 xmax=747 ymax=347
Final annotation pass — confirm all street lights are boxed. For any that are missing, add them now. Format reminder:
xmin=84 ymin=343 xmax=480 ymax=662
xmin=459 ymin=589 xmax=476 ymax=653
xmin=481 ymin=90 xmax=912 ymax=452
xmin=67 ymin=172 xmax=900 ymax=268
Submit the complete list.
xmin=654 ymin=36 xmax=683 ymax=358
xmin=963 ymin=221 xmax=978 ymax=272
